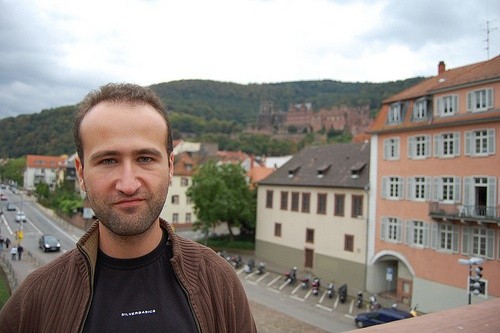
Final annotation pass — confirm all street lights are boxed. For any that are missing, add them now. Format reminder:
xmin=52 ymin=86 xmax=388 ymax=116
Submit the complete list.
xmin=458 ymin=256 xmax=485 ymax=305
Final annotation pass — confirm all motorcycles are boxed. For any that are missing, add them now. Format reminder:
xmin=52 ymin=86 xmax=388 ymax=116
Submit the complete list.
xmin=286 ymin=266 xmax=381 ymax=311
xmin=216 ymin=249 xmax=266 ymax=275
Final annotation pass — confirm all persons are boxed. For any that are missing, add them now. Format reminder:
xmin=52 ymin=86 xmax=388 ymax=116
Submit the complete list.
xmin=12 ymin=245 xmax=23 ymax=261
xmin=0 ymin=83 xmax=259 ymax=333
xmin=5 ymin=238 xmax=11 ymax=248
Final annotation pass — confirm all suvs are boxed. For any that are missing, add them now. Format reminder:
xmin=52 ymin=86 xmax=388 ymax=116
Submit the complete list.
xmin=354 ymin=308 xmax=414 ymax=328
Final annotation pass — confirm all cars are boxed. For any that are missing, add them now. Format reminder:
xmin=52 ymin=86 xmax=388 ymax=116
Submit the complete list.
xmin=9 ymin=184 xmax=21 ymax=196
xmin=7 ymin=202 xmax=17 ymax=211
xmin=0 ymin=183 xmax=7 ymax=189
xmin=38 ymin=233 xmax=61 ymax=253
xmin=0 ymin=193 xmax=9 ymax=201
xmin=14 ymin=210 xmax=27 ymax=222
